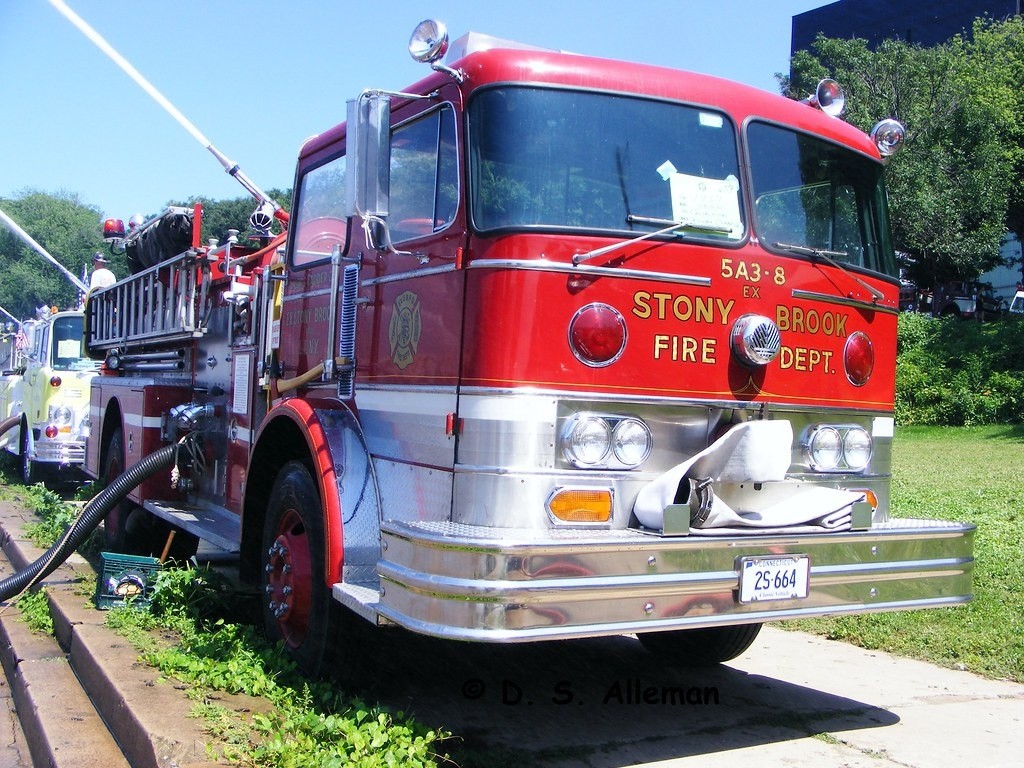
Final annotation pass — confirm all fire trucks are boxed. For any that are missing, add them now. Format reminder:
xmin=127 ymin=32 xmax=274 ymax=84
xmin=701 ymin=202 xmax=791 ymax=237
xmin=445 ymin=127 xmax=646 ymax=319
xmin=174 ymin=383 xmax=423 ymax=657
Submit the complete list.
xmin=0 ymin=302 xmax=108 ymax=490
xmin=84 ymin=16 xmax=977 ymax=690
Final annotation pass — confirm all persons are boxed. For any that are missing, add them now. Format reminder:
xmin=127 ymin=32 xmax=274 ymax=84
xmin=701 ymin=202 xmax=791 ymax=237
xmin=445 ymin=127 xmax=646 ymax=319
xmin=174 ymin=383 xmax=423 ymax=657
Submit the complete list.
xmin=90 ymin=251 xmax=117 ymax=340
xmin=233 ymin=300 xmax=252 ymax=342
xmin=1013 ymin=280 xmax=1024 ymax=308
xmin=933 ymin=283 xmax=987 ymax=324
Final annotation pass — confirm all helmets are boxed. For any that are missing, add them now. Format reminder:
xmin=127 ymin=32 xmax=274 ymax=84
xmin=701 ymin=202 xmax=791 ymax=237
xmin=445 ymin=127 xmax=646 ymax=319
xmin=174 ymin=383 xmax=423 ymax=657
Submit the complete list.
xmin=51 ymin=307 xmax=58 ymax=313
xmin=92 ymin=250 xmax=112 ymax=263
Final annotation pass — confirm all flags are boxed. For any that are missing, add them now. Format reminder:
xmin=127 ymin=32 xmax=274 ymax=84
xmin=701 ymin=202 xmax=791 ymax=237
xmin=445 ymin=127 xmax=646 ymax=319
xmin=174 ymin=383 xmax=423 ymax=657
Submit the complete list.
xmin=15 ymin=324 xmax=30 ymax=350
xmin=78 ymin=265 xmax=90 ymax=309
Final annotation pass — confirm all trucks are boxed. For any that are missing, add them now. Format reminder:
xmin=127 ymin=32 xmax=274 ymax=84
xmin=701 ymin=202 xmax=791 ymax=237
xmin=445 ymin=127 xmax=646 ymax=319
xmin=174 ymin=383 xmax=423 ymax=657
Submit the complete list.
xmin=893 ymin=250 xmax=1024 ymax=322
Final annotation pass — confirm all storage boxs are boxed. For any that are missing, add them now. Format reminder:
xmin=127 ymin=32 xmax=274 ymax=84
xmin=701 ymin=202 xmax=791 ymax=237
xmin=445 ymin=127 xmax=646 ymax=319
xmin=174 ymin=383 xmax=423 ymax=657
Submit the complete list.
xmin=97 ymin=551 xmax=162 ymax=611
